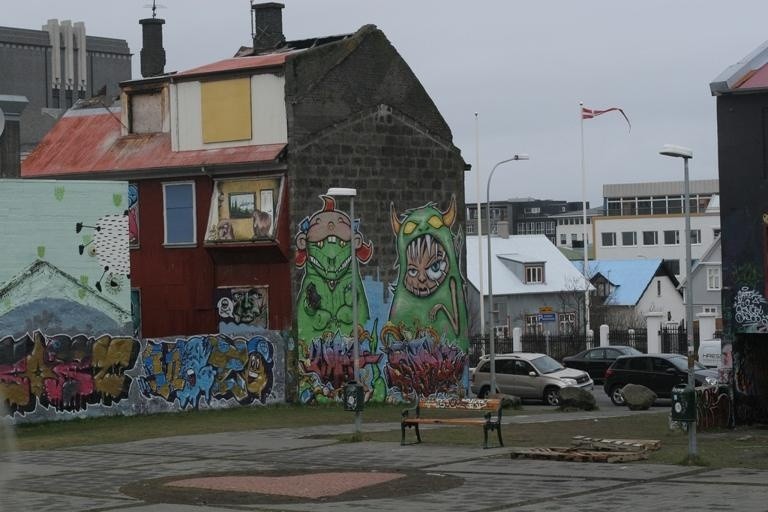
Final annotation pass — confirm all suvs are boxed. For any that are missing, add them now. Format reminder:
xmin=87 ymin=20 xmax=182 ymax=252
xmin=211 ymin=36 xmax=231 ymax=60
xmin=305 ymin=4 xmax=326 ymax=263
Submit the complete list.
xmin=469 ymin=350 xmax=594 ymax=408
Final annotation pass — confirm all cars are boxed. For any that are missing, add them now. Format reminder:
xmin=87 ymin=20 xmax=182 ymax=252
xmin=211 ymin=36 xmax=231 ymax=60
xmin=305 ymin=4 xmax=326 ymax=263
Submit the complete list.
xmin=561 ymin=345 xmax=644 ymax=383
xmin=601 ymin=352 xmax=718 ymax=407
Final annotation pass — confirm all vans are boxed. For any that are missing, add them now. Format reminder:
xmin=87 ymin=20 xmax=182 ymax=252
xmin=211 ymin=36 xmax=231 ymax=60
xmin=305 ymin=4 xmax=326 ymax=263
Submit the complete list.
xmin=695 ymin=338 xmax=721 ymax=366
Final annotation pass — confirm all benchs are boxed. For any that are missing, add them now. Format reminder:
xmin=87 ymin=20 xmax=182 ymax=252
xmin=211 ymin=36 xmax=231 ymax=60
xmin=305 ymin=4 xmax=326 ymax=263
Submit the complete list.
xmin=400 ymin=397 xmax=505 ymax=450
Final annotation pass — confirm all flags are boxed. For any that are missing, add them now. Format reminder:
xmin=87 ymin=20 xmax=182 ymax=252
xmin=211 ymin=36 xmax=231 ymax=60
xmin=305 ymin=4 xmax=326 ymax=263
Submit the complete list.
xmin=583 ymin=108 xmax=630 ymax=128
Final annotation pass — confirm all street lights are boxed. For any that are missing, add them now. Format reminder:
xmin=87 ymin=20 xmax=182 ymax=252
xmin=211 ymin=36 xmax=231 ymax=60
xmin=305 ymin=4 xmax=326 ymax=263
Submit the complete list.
xmin=655 ymin=143 xmax=697 ymax=388
xmin=323 ymin=185 xmax=364 ymax=437
xmin=485 ymin=153 xmax=530 ymax=398
